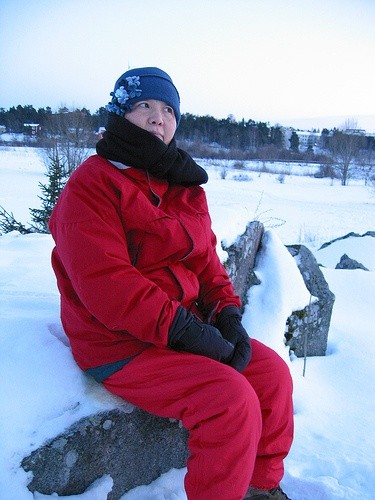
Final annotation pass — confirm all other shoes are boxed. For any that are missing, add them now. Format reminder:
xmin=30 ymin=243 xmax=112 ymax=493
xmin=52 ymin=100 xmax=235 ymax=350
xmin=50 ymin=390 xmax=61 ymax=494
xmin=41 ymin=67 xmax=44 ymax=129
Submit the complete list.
xmin=243 ymin=487 xmax=292 ymax=500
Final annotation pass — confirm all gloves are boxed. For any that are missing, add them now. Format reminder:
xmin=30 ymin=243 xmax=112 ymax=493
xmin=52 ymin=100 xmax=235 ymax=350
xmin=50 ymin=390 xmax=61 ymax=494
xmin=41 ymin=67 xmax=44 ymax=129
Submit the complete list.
xmin=213 ymin=305 xmax=252 ymax=372
xmin=167 ymin=305 xmax=235 ymax=361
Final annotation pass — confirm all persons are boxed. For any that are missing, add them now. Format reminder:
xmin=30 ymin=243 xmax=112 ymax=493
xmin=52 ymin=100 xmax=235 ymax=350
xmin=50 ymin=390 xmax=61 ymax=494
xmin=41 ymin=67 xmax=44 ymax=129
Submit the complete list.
xmin=48 ymin=67 xmax=294 ymax=500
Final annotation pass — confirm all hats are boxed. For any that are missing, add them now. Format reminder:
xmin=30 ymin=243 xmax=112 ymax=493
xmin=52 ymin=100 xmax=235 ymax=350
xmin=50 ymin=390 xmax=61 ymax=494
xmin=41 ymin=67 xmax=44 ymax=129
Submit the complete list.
xmin=106 ymin=67 xmax=181 ymax=129
